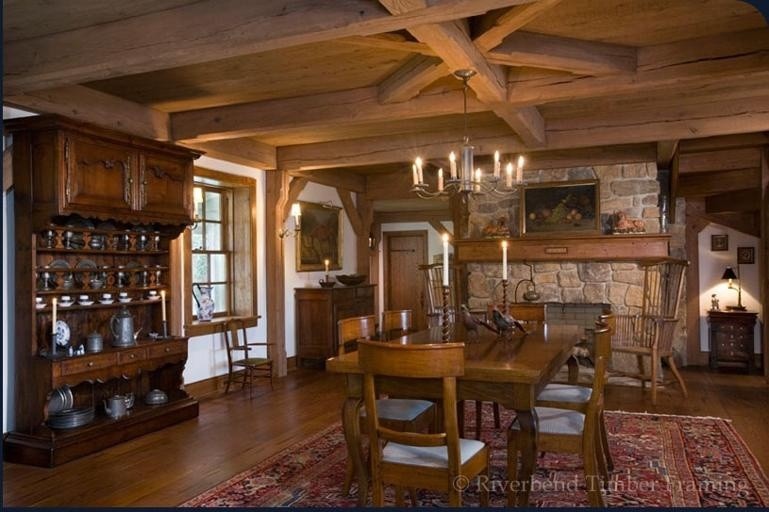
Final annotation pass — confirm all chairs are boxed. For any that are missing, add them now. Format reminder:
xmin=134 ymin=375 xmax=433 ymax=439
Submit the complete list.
xmin=339 ymin=304 xmax=616 ymax=510
xmin=601 ymin=256 xmax=692 ymax=407
xmin=223 ymin=319 xmax=275 ymax=400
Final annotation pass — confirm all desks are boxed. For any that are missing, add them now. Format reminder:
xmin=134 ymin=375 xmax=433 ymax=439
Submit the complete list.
xmin=325 ymin=322 xmax=588 ymax=508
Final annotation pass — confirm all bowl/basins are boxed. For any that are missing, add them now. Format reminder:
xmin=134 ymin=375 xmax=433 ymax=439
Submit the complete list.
xmin=335 ymin=273 xmax=368 ymax=286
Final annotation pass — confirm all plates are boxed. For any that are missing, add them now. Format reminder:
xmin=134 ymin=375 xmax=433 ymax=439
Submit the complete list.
xmin=37 ymin=258 xmax=146 ymax=289
xmin=33 ymin=290 xmax=161 ymax=312
xmin=55 ymin=320 xmax=72 ymax=347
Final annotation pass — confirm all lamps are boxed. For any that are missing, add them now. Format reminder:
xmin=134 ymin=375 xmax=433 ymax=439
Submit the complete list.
xmin=410 ymin=69 xmax=526 ymax=204
xmin=368 ymin=233 xmax=376 ymax=248
xmin=185 ymin=187 xmax=203 ymax=231
xmin=279 ymin=203 xmax=302 ymax=238
xmin=722 ymin=265 xmax=746 ymax=311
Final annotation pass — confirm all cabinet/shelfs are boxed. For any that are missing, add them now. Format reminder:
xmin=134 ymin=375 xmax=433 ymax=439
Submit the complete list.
xmin=295 ymin=284 xmax=379 ymax=368
xmin=707 ymin=309 xmax=760 ymax=374
xmin=0 ymin=113 xmax=207 ymax=468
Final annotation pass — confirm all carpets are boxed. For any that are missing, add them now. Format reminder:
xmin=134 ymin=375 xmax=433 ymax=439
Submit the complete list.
xmin=178 ymin=390 xmax=769 ymax=510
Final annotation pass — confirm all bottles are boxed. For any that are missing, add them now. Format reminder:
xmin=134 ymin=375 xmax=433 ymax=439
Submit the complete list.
xmin=85 ymin=329 xmax=105 ymax=355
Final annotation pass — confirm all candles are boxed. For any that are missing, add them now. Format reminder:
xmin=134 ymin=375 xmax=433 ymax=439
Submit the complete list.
xmin=441 ymin=232 xmax=449 ymax=285
xmin=501 ymin=239 xmax=509 ymax=279
xmin=324 ymin=259 xmax=330 ymax=275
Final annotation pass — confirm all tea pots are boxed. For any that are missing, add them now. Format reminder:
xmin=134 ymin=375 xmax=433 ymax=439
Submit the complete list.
xmin=101 ymin=393 xmax=128 ymax=421
xmin=109 ymin=303 xmax=139 ymax=348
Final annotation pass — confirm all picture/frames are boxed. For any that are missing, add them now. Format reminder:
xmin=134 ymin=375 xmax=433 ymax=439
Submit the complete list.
xmin=519 ymin=178 xmax=602 ymax=240
xmin=296 ymin=199 xmax=344 ymax=272
xmin=712 ymin=234 xmax=728 ymax=251
xmin=738 ymin=247 xmax=754 ymax=264
